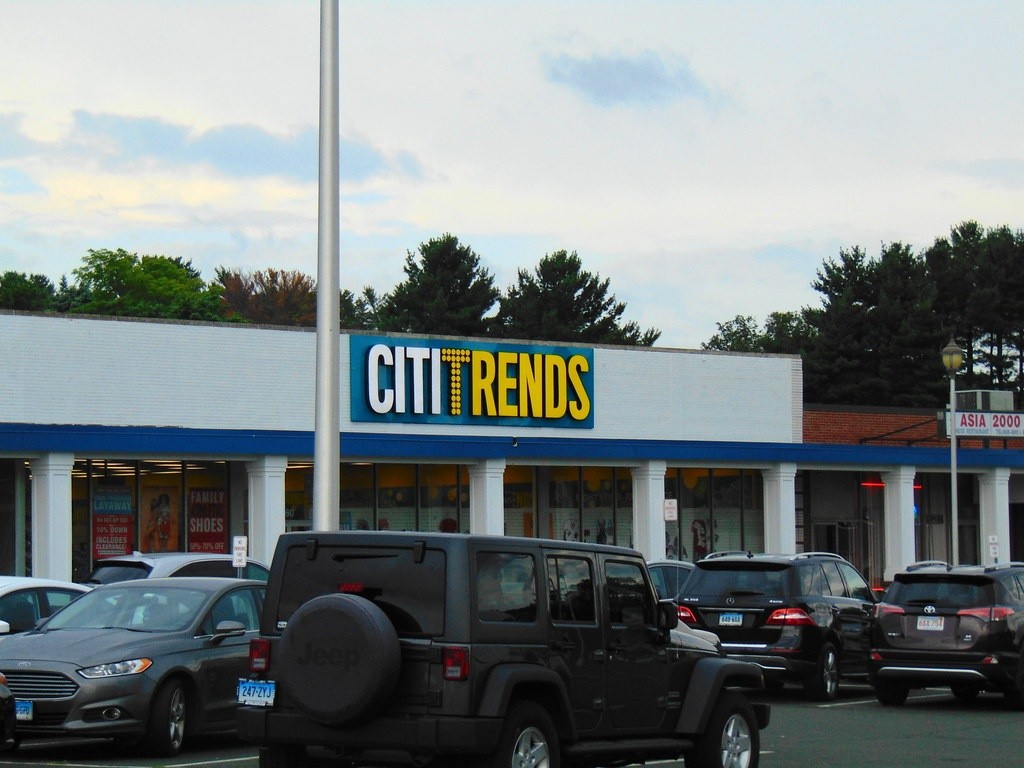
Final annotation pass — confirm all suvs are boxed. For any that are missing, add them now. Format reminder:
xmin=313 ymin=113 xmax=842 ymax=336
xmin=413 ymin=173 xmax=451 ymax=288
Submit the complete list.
xmin=84 ymin=551 xmax=270 ymax=598
xmin=866 ymin=560 xmax=1024 ymax=711
xmin=237 ymin=525 xmax=773 ymax=768
xmin=667 ymin=550 xmax=880 ymax=703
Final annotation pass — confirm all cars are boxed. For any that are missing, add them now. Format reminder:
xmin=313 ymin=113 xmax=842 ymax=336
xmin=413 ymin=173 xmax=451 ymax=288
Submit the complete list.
xmin=0 ymin=573 xmax=191 ymax=636
xmin=634 ymin=559 xmax=695 ymax=621
xmin=0 ymin=576 xmax=269 ymax=760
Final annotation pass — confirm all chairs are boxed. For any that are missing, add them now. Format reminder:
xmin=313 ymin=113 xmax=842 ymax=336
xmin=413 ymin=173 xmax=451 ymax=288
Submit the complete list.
xmin=779 ymin=571 xmax=788 ymax=591
xmin=947 ymin=583 xmax=970 ymax=595
xmin=16 ymin=602 xmax=34 ymax=627
xmin=143 ymin=604 xmax=171 ymax=626
xmin=213 ymin=597 xmax=250 ymax=630
xmin=746 ymin=571 xmax=768 ymax=589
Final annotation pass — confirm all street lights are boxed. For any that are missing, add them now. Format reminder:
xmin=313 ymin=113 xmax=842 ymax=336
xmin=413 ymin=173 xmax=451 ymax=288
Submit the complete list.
xmin=941 ymin=332 xmax=966 ymax=566
